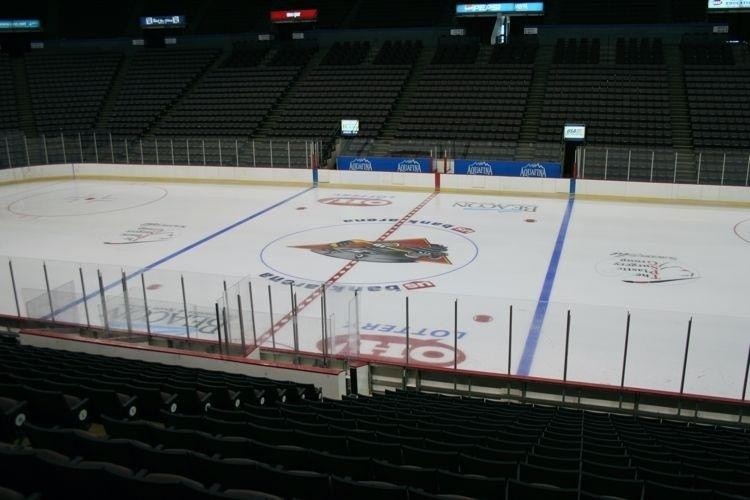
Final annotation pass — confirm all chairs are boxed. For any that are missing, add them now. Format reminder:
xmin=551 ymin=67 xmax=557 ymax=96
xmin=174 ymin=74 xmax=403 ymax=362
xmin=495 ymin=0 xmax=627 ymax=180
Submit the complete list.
xmin=1 ymin=344 xmax=323 ymax=499
xmin=324 ymin=385 xmax=749 ymax=500
xmin=0 ymin=62 xmax=749 ymax=183
